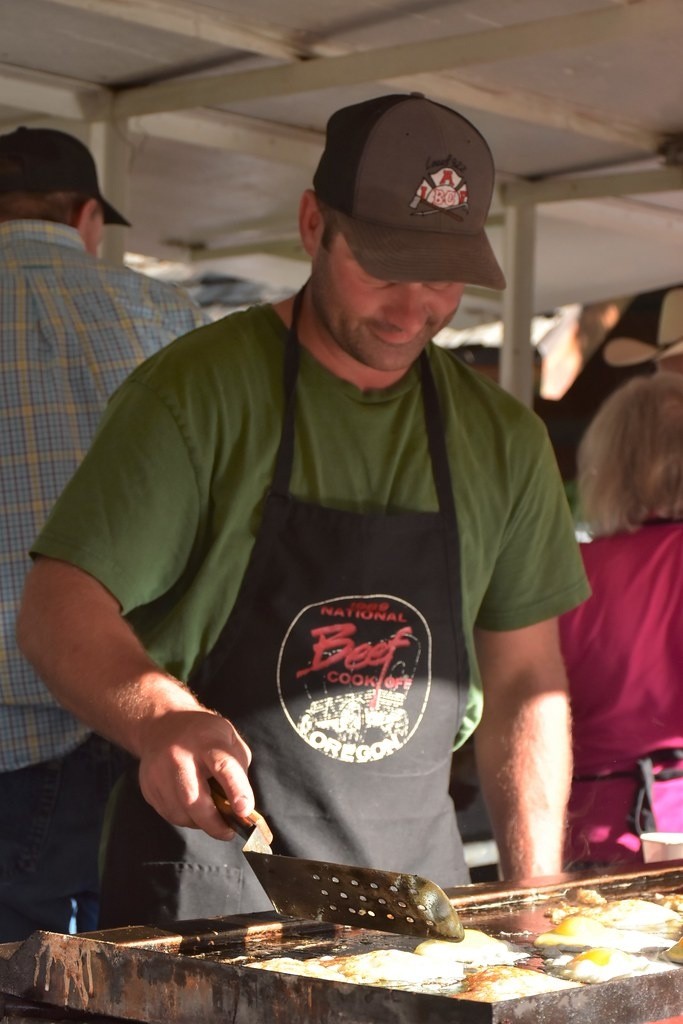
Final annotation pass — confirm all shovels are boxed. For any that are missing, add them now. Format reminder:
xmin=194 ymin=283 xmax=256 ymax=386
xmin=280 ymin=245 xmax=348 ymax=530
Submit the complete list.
xmin=210 ymin=791 xmax=463 ymax=944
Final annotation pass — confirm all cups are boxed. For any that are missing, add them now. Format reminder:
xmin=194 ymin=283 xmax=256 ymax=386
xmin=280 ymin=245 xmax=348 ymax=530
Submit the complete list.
xmin=640 ymin=832 xmax=683 ymax=863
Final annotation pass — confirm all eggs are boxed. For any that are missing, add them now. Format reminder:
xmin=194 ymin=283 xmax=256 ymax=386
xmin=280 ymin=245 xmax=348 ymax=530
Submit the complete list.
xmin=242 ymin=887 xmax=683 ymax=1002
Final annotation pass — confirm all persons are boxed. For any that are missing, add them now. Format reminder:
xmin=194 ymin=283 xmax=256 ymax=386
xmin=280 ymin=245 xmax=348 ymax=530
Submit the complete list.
xmin=559 ymin=368 xmax=681 ymax=870
xmin=17 ymin=92 xmax=597 ymax=926
xmin=1 ymin=123 xmax=219 ymax=941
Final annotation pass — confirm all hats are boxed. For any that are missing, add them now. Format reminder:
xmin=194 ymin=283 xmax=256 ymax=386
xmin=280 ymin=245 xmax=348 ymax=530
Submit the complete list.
xmin=311 ymin=90 xmax=509 ymax=292
xmin=1 ymin=126 xmax=132 ymax=226
